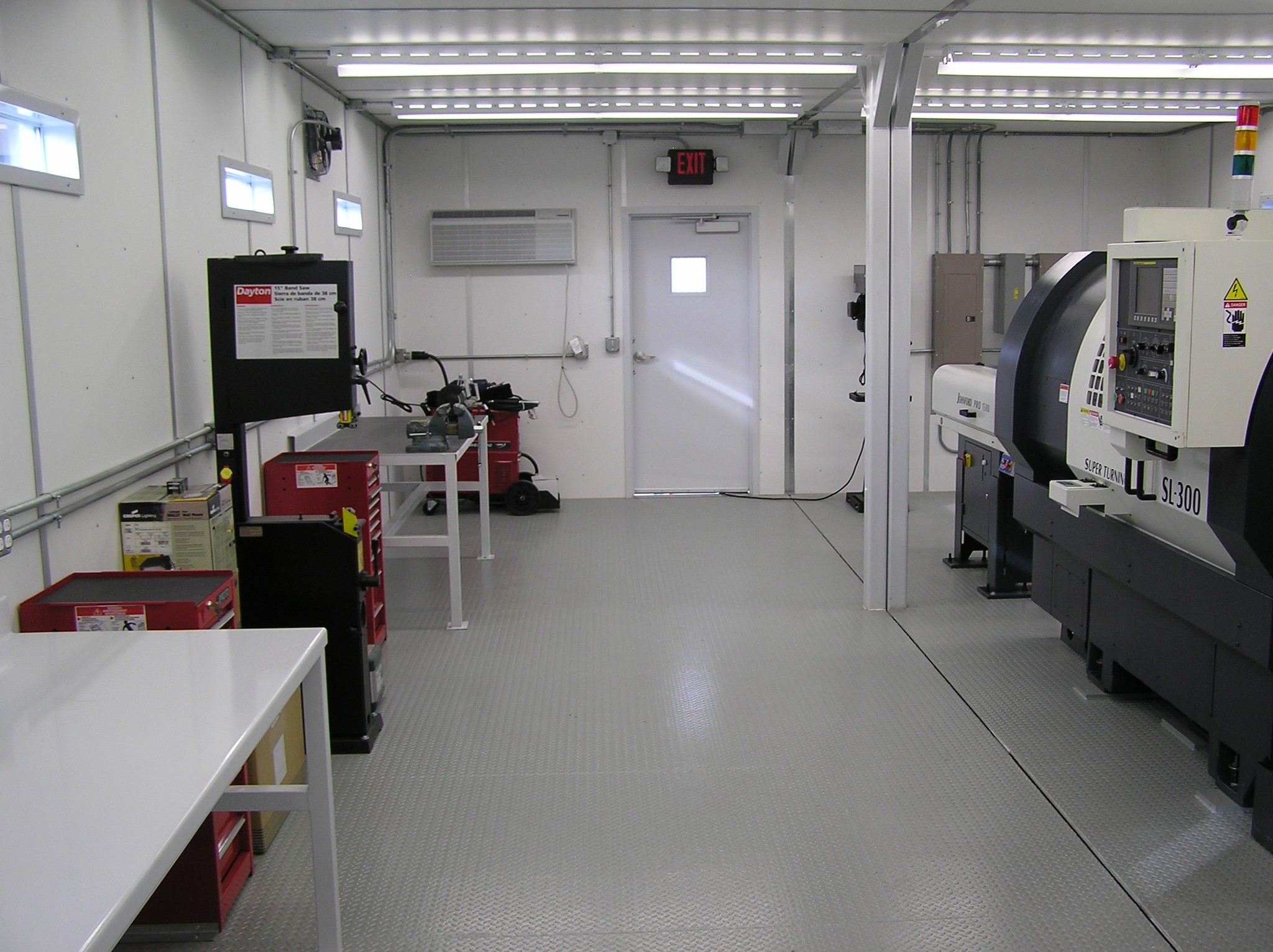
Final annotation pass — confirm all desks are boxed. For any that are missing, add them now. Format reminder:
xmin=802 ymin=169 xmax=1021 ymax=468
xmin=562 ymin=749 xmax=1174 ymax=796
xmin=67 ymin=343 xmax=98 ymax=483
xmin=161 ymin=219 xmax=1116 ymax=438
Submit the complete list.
xmin=1 ymin=628 xmax=345 ymax=952
xmin=296 ymin=415 xmax=497 ymax=634
